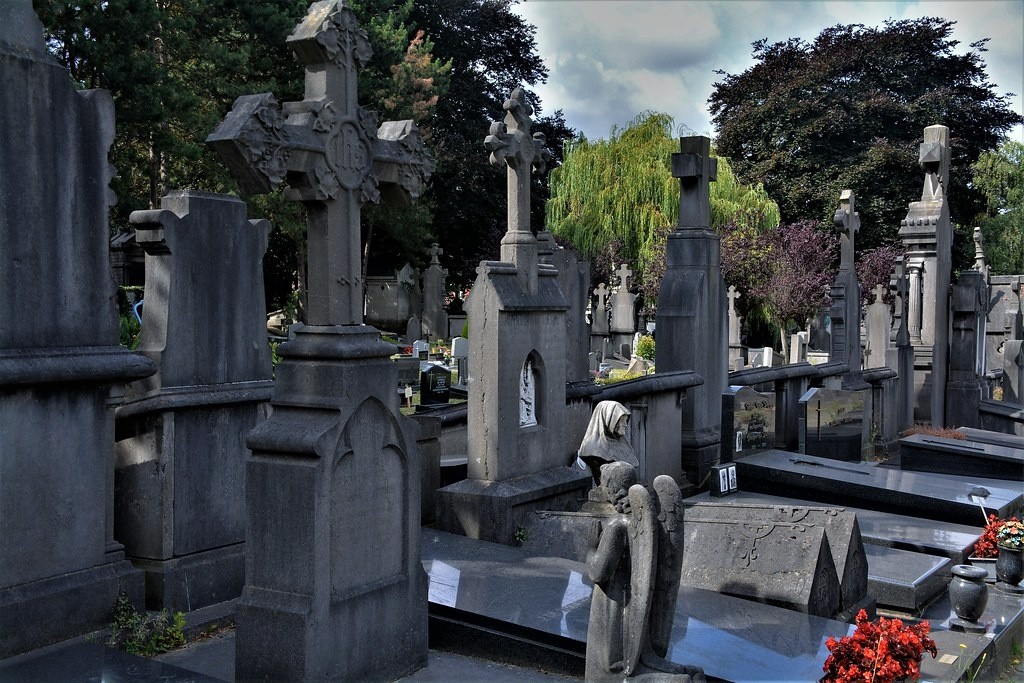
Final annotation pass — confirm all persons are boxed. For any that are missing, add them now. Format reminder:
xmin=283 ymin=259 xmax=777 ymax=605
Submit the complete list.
xmin=577 ymin=401 xmax=639 ymax=494
xmin=585 ymin=460 xmax=639 ymax=683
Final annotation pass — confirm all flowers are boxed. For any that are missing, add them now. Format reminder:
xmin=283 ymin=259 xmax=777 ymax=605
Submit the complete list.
xmin=997 ymin=517 xmax=1024 ymax=550
xmin=974 ymin=515 xmax=1006 ymax=557
xmin=820 ymin=605 xmax=939 ymax=683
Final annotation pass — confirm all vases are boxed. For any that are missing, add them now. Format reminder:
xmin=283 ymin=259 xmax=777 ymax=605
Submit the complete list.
xmin=949 ymin=564 xmax=989 ymax=634
xmin=997 ymin=544 xmax=1024 ymax=593
xmin=968 ymin=553 xmax=998 ymax=584
